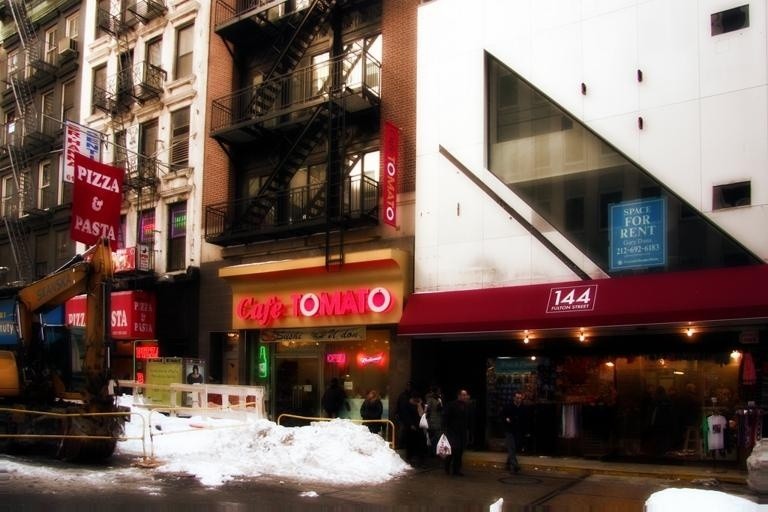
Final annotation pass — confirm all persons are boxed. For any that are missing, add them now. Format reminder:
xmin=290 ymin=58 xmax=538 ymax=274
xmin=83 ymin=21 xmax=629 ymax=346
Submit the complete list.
xmin=392 ymin=381 xmax=446 ymax=462
xmin=443 ymin=387 xmax=469 ymax=477
xmin=187 ymin=364 xmax=203 ymax=385
xmin=498 ymin=390 xmax=532 ymax=474
xmin=321 ymin=377 xmax=350 ymax=418
xmin=359 ymin=389 xmax=383 ymax=434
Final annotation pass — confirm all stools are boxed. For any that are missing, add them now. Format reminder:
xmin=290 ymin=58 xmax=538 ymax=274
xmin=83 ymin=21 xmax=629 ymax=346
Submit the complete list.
xmin=683 ymin=426 xmax=698 ymax=450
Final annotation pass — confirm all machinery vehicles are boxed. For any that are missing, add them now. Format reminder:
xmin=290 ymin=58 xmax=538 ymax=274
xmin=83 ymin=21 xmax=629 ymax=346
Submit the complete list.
xmin=0 ymin=236 xmax=130 ymax=462
